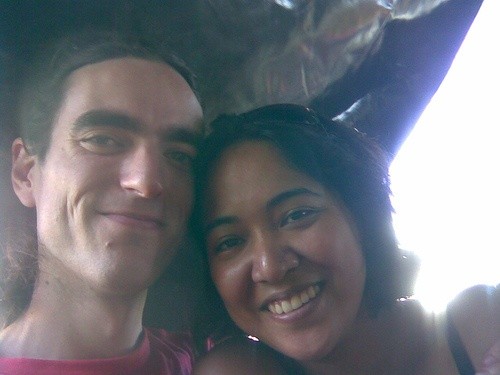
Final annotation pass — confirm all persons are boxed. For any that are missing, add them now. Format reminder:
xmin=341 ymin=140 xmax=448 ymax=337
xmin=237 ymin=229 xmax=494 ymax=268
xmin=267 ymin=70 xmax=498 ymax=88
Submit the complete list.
xmin=191 ymin=103 xmax=500 ymax=375
xmin=0 ymin=30 xmax=500 ymax=375
